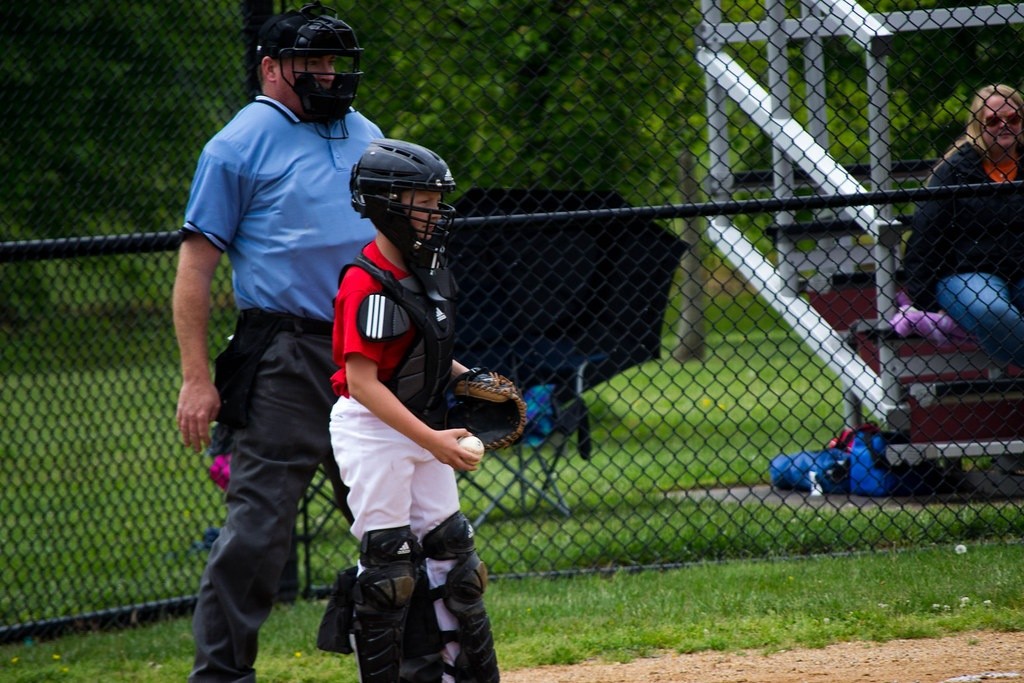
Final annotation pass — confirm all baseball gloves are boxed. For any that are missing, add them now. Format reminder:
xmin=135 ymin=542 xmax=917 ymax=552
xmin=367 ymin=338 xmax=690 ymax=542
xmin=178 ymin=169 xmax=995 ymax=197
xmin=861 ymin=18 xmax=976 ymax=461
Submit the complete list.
xmin=446 ymin=366 xmax=527 ymax=450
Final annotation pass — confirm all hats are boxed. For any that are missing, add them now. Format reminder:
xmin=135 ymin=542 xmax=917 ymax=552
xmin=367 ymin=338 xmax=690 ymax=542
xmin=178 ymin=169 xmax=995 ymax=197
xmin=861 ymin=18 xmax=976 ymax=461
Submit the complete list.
xmin=256 ymin=12 xmax=352 ymax=58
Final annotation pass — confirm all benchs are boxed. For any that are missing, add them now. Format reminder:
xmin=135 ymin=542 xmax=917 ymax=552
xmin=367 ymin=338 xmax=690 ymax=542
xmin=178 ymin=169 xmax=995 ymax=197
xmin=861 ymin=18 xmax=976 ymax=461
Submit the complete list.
xmin=730 ymin=166 xmax=1022 ymax=466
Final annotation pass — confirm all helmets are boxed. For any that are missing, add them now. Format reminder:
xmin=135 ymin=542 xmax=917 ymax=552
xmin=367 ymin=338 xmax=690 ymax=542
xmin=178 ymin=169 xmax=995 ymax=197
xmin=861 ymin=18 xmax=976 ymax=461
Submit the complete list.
xmin=350 ymin=139 xmax=454 ymax=275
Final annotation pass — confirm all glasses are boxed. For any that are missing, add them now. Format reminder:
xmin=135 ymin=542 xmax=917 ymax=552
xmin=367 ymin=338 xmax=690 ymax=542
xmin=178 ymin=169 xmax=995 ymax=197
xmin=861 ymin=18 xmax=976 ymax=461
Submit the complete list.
xmin=981 ymin=113 xmax=1022 ymax=127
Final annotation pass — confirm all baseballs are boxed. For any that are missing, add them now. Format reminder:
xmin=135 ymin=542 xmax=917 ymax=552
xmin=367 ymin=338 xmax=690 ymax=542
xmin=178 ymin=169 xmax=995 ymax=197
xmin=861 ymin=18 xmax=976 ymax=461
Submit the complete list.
xmin=457 ymin=436 xmax=485 ymax=464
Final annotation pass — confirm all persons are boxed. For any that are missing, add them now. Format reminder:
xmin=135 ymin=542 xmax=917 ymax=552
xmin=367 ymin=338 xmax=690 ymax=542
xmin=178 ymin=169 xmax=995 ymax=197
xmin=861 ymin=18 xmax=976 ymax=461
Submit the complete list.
xmin=170 ymin=0 xmax=529 ymax=682
xmin=327 ymin=136 xmax=502 ymax=683
xmin=901 ymin=82 xmax=1024 ymax=373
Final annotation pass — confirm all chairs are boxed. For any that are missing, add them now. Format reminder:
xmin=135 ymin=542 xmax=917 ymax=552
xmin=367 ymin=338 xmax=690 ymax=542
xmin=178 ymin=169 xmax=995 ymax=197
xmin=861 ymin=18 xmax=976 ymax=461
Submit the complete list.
xmin=459 ymin=363 xmax=590 ymax=530
